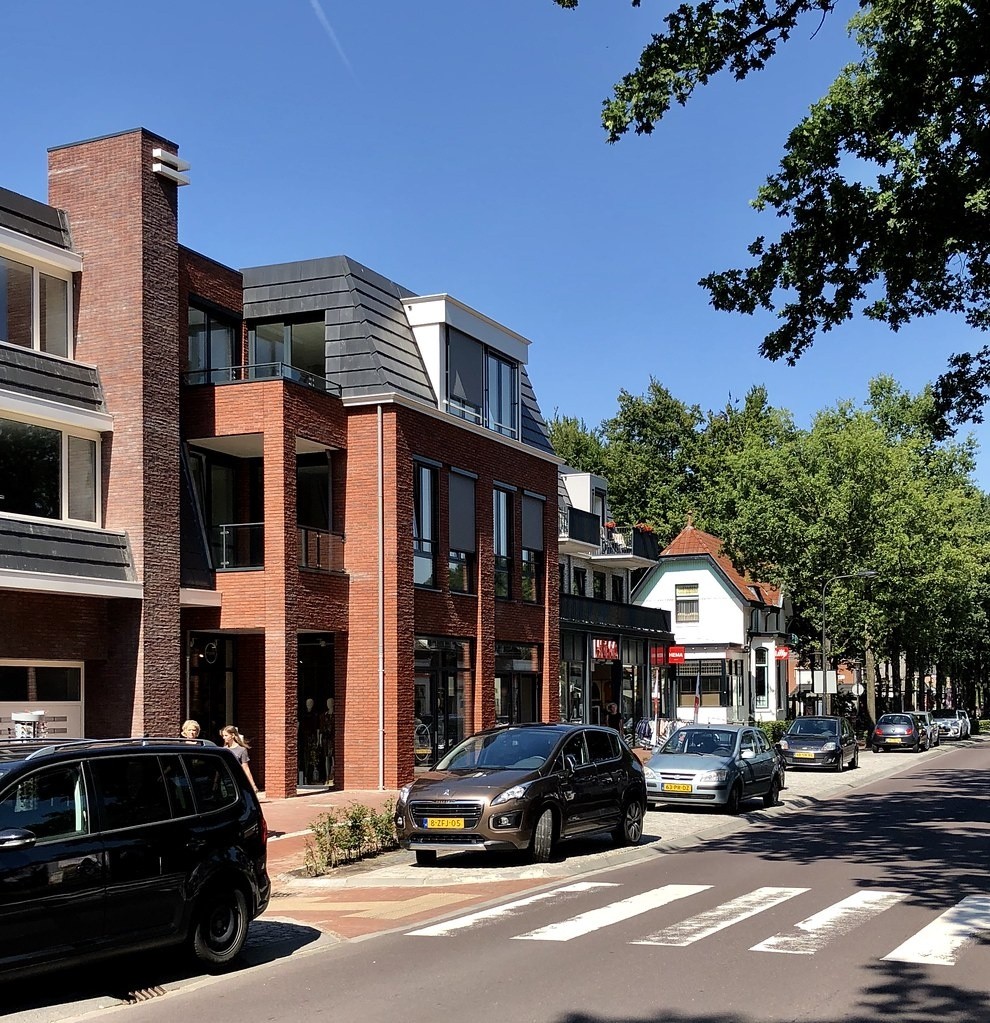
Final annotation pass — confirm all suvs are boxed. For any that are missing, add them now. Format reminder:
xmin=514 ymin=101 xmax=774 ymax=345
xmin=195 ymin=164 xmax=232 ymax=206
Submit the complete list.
xmin=0 ymin=735 xmax=271 ymax=992
xmin=393 ymin=722 xmax=650 ymax=867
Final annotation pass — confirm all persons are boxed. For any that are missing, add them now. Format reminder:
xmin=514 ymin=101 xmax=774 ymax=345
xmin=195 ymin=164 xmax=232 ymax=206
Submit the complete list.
xmin=297 ymin=699 xmax=335 ymax=785
xmin=220 ymin=725 xmax=258 ymax=794
xmin=180 ymin=720 xmax=201 ymax=746
xmin=606 ymin=704 xmax=623 ymax=737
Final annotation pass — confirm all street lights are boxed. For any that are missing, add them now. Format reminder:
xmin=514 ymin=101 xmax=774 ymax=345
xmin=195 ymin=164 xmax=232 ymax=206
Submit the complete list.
xmin=823 ymin=569 xmax=881 ymax=716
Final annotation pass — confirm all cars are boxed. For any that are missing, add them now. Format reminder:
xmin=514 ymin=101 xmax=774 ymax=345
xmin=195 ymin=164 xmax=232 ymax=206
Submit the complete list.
xmin=869 ymin=713 xmax=928 ymax=753
xmin=642 ymin=722 xmax=786 ymax=813
xmin=905 ymin=711 xmax=941 ymax=749
xmin=776 ymin=714 xmax=861 ymax=772
xmin=930 ymin=709 xmax=973 ymax=741
